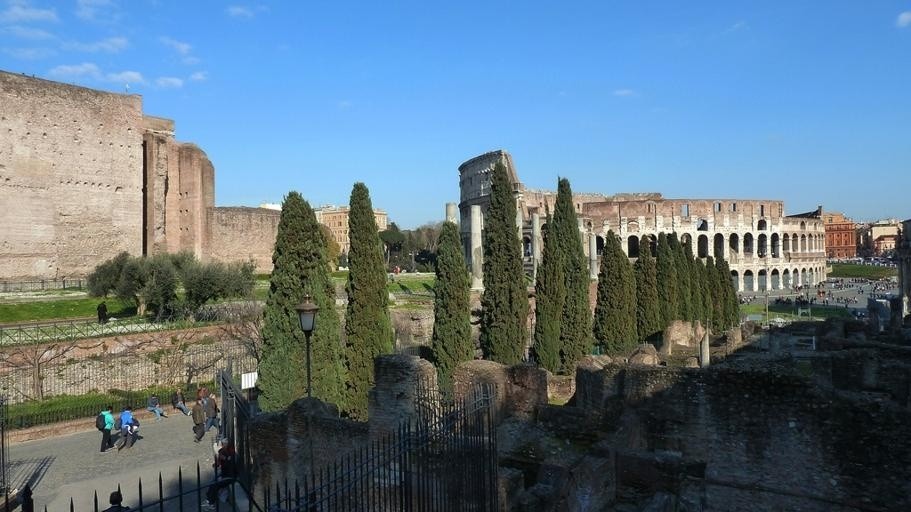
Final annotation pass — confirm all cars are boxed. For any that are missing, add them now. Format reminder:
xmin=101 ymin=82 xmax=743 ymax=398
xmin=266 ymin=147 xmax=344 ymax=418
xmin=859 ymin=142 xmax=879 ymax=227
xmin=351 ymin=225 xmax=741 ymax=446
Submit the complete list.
xmin=828 ymin=256 xmax=897 ymax=267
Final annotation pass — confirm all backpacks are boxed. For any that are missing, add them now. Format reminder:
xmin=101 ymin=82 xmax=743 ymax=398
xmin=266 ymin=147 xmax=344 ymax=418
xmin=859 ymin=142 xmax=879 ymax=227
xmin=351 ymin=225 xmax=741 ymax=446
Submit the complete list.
xmin=95 ymin=412 xmax=109 ymax=431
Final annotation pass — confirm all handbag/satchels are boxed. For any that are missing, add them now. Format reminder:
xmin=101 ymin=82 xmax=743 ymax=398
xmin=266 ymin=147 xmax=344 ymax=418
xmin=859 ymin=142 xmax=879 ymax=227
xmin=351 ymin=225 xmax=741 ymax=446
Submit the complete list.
xmin=114 ymin=414 xmax=123 ymax=431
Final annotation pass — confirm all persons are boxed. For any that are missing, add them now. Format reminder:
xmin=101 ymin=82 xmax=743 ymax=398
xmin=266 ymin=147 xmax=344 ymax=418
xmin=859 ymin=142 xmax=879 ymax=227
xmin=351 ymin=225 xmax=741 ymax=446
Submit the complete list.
xmin=100 ymin=490 xmax=131 ymax=511
xmin=173 ymin=386 xmax=193 ymax=416
xmin=146 ymin=394 xmax=162 ymax=421
xmin=115 ymin=405 xmax=139 ymax=451
xmin=192 ymin=385 xmax=222 ymax=444
xmin=97 ymin=300 xmax=109 ymax=324
xmin=200 ymin=436 xmax=236 ymax=511
xmin=98 ymin=405 xmax=118 ymax=453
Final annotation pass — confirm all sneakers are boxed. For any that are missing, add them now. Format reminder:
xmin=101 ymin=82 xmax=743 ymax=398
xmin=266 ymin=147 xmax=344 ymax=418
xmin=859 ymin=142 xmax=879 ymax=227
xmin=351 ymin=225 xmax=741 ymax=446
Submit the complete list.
xmin=193 ymin=434 xmax=204 ymax=444
xmin=99 ymin=444 xmax=119 ymax=454
xmin=187 ymin=408 xmax=194 ymax=417
xmin=200 ymin=499 xmax=217 ymax=512
xmin=156 ymin=412 xmax=168 ymax=423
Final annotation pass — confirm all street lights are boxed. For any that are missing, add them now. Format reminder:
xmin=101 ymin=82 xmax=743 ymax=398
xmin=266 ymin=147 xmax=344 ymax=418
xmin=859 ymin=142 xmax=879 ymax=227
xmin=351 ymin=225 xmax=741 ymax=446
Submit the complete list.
xmin=292 ymin=292 xmax=320 ymax=511
xmin=758 ymin=248 xmax=776 ymax=293
